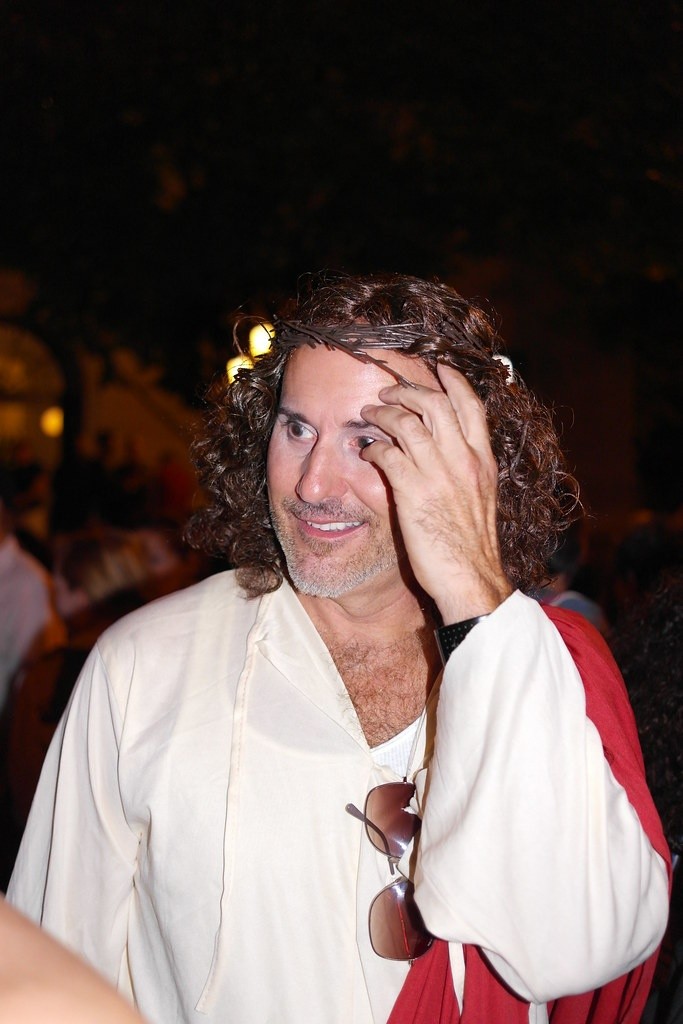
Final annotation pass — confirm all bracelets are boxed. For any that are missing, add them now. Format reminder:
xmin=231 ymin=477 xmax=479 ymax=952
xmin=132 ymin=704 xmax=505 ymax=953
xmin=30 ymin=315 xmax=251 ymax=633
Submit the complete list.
xmin=434 ymin=611 xmax=491 ymax=672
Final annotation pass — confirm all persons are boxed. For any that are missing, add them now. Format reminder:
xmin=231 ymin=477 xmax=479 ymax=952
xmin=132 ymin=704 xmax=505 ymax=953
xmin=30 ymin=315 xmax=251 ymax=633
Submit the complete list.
xmin=1 ymin=278 xmax=672 ymax=1024
xmin=0 ymin=498 xmax=68 ymax=719
xmin=603 ymin=564 xmax=682 ymax=1024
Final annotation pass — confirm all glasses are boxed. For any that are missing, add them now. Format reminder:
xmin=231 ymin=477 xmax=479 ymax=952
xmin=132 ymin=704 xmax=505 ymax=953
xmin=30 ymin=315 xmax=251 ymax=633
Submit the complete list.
xmin=345 ymin=776 xmax=436 ymax=965
